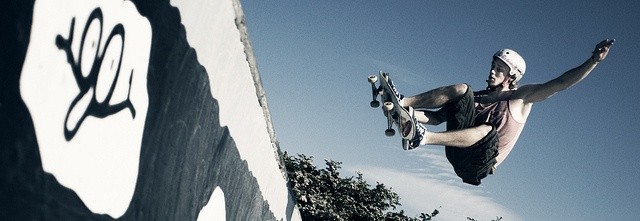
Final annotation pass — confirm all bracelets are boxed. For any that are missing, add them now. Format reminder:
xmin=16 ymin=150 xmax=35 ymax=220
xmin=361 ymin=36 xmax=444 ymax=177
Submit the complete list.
xmin=591 ymin=55 xmax=600 ymax=65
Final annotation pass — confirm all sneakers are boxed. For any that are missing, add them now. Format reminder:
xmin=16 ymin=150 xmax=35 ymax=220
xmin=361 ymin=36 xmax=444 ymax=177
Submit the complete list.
xmin=402 ymin=106 xmax=426 ymax=150
xmin=384 ymin=73 xmax=405 ymax=106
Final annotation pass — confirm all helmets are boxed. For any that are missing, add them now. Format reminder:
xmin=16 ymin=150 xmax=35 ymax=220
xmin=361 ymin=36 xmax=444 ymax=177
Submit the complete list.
xmin=493 ymin=48 xmax=526 ymax=86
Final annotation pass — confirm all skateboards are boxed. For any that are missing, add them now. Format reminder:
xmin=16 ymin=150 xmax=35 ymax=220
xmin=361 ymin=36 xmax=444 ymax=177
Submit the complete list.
xmin=367 ymin=71 xmax=415 ymax=140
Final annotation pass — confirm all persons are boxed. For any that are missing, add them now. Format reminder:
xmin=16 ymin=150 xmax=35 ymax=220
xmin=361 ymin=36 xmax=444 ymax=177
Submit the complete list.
xmin=382 ymin=38 xmax=616 ymax=185
